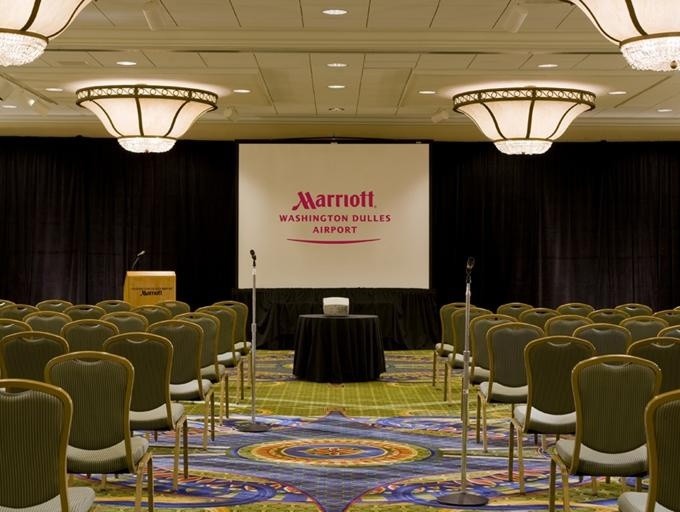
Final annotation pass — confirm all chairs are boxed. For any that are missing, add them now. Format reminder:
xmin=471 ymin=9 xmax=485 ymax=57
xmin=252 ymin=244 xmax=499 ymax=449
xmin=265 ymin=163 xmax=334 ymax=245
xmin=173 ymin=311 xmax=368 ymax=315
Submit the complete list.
xmin=1 ymin=331 xmax=71 ymax=384
xmin=432 ymin=300 xmax=680 ymax=386
xmin=459 ymin=315 xmax=520 ymax=425
xmin=545 ymin=352 xmax=661 ymax=512
xmin=1 ymin=375 xmax=101 ymax=511
xmin=1 ymin=299 xmax=192 ymax=349
xmin=42 ymin=350 xmax=158 ymax=512
xmin=196 ymin=305 xmax=248 ymax=407
xmin=474 ymin=323 xmax=545 ymax=455
xmin=614 ymin=386 xmax=680 ymax=512
xmin=102 ymin=331 xmax=192 ymax=492
xmin=442 ymin=308 xmax=493 ymax=403
xmin=173 ymin=313 xmax=233 ymax=428
xmin=212 ymin=300 xmax=255 ymax=386
xmin=506 ymin=335 xmax=598 ymax=495
xmin=148 ymin=320 xmax=217 ymax=452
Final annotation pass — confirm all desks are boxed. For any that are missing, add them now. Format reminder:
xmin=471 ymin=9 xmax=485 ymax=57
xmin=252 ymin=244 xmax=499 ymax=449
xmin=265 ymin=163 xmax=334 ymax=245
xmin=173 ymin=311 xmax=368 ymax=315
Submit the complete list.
xmin=292 ymin=315 xmax=387 ymax=384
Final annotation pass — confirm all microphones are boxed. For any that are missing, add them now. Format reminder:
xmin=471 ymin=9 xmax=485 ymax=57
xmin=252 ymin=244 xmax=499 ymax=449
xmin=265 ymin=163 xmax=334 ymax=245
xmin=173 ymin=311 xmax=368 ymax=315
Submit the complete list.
xmin=466 ymin=257 xmax=475 ymax=274
xmin=250 ymin=249 xmax=256 ymax=260
xmin=137 ymin=250 xmax=145 ymax=257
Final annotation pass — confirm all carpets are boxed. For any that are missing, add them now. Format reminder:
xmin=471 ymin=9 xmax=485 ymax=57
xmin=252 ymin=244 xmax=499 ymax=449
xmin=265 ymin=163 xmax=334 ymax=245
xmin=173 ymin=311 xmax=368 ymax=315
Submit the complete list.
xmin=66 ymin=405 xmax=652 ymax=512
xmin=223 ymin=352 xmax=463 ymax=385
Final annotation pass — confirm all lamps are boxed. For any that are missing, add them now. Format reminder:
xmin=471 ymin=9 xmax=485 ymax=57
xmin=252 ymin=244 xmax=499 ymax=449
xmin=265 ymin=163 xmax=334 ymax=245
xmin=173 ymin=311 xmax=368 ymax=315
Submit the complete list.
xmin=1 ymin=0 xmax=93 ymax=70
xmin=74 ymin=82 xmax=219 ymax=156
xmin=563 ymin=0 xmax=680 ymax=72
xmin=451 ymin=86 xmax=599 ymax=158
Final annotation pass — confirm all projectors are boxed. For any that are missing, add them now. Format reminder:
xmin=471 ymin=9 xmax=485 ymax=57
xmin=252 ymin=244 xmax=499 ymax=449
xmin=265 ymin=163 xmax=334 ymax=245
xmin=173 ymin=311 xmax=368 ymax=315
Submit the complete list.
xmin=323 ymin=298 xmax=350 ymax=317
